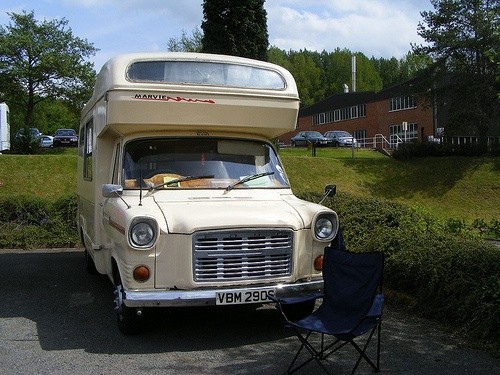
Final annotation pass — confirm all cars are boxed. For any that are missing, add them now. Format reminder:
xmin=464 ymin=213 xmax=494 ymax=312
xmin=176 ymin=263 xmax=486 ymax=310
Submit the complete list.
xmin=53 ymin=129 xmax=78 ymax=148
xmin=289 ymin=131 xmax=332 ymax=148
xmin=15 ymin=128 xmax=54 ymax=148
xmin=322 ymin=131 xmax=360 ymax=147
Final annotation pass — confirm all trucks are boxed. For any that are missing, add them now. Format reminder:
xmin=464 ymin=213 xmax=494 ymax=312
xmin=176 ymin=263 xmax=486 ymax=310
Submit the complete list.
xmin=77 ymin=50 xmax=340 ymax=337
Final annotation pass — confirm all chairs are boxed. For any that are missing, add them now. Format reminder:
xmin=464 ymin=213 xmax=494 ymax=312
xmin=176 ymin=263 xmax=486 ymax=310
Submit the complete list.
xmin=267 ymin=246 xmax=386 ymax=375
